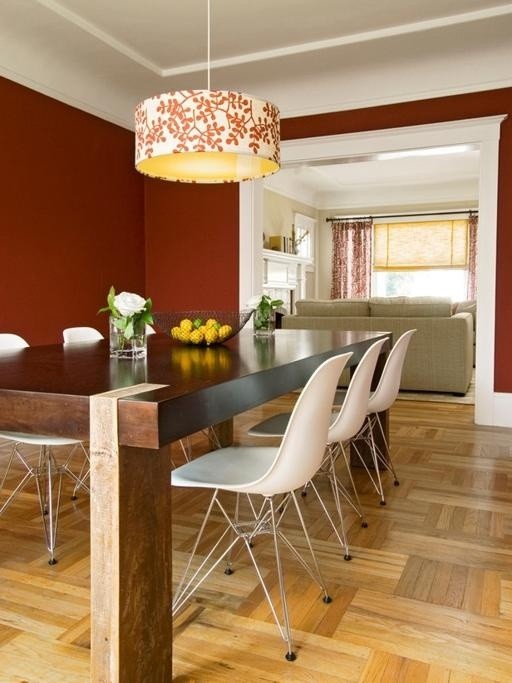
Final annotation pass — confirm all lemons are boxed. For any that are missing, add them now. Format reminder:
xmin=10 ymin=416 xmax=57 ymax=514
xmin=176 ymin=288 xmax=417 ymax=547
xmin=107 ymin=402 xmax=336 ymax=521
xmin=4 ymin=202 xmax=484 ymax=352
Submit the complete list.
xmin=171 ymin=319 xmax=232 ymax=345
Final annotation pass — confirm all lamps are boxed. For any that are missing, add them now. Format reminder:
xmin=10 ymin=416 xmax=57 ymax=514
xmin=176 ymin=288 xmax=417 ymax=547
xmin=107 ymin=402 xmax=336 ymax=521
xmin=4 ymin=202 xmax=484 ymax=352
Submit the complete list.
xmin=132 ymin=0 xmax=284 ymax=186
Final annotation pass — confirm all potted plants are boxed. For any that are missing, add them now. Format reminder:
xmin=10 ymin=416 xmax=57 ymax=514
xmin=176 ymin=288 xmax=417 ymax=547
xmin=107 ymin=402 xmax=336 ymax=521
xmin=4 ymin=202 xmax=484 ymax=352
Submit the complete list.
xmin=250 ymin=293 xmax=285 ymax=330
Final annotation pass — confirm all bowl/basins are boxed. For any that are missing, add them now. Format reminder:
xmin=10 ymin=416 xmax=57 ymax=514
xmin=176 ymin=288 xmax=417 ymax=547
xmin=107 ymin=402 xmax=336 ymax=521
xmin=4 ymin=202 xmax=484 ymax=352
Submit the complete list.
xmin=151 ymin=308 xmax=256 ymax=344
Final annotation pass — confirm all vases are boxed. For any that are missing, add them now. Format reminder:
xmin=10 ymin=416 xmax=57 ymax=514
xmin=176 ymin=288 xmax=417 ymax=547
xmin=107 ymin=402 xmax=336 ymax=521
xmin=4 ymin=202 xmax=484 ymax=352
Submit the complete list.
xmin=106 ymin=313 xmax=149 ymax=359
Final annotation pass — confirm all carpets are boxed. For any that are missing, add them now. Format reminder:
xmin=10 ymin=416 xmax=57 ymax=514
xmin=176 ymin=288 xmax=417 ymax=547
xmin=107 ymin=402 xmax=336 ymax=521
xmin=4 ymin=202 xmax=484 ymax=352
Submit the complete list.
xmin=286 ymin=367 xmax=477 ymax=405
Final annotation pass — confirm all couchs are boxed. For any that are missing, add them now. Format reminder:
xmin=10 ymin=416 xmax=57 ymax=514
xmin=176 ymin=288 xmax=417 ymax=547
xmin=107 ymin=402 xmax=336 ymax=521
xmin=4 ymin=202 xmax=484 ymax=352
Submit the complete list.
xmin=279 ymin=296 xmax=475 ymax=398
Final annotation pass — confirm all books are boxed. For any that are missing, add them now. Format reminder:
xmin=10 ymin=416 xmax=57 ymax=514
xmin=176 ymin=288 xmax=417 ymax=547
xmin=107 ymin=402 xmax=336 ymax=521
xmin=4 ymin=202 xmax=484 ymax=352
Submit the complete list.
xmin=270 ymin=236 xmax=293 ymax=253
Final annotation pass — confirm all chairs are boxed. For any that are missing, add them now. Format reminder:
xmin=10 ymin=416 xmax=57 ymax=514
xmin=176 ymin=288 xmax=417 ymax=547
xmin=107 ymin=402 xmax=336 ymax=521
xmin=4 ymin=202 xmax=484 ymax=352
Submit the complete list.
xmin=112 ymin=321 xmax=224 ymax=471
xmin=247 ymin=336 xmax=388 ymax=561
xmin=169 ymin=349 xmax=354 ymax=664
xmin=303 ymin=330 xmax=419 ymax=510
xmin=53 ymin=326 xmax=106 ymax=502
xmin=0 ymin=331 xmax=89 ymax=568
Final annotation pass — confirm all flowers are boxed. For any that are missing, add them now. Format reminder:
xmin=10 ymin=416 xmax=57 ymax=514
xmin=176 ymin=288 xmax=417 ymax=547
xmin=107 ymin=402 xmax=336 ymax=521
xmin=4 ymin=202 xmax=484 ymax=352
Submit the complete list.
xmin=93 ymin=282 xmax=156 ymax=352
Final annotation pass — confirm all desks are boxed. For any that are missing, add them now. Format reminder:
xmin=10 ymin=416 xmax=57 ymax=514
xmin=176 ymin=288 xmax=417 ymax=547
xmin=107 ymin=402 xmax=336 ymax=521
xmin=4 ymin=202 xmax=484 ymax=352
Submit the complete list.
xmin=0 ymin=323 xmax=394 ymax=682
